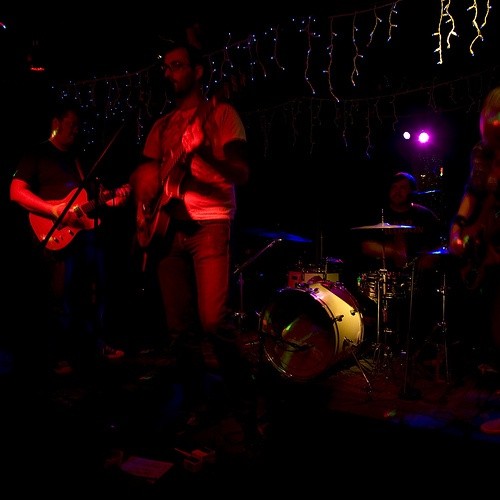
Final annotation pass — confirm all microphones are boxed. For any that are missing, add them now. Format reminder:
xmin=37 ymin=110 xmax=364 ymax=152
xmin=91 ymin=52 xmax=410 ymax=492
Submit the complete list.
xmin=408 ymin=188 xmax=443 ymax=197
xmin=296 ymin=342 xmax=318 ymax=352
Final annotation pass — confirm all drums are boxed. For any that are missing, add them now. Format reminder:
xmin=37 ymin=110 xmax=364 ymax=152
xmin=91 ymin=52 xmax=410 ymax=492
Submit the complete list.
xmin=285 ymin=270 xmax=342 ymax=288
xmin=361 ymin=268 xmax=419 ymax=302
xmin=259 ymin=277 xmax=365 ymax=386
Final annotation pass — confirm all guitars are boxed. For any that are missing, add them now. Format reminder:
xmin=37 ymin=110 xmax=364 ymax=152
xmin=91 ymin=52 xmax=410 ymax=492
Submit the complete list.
xmin=27 ymin=174 xmax=136 ymax=261
xmin=133 ymin=83 xmax=236 ymax=254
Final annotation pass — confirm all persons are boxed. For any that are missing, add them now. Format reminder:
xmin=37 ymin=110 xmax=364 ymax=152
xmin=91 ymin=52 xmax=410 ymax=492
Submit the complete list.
xmin=9 ymin=105 xmax=130 ymax=375
xmin=449 ymin=87 xmax=500 ymax=392
xmin=364 ymin=171 xmax=439 ymax=357
xmin=136 ymin=41 xmax=250 ymax=386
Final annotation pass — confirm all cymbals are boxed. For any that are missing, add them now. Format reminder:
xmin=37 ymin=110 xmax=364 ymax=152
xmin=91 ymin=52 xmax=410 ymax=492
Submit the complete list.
xmin=415 ymin=249 xmax=466 ymax=256
xmin=349 ymin=224 xmax=425 ymax=233
xmin=322 ymin=256 xmax=353 ymax=265
xmin=250 ymin=230 xmax=311 ymax=242
